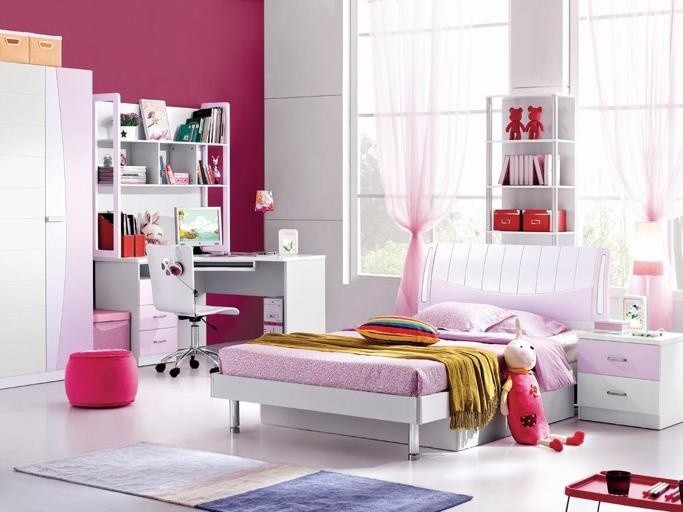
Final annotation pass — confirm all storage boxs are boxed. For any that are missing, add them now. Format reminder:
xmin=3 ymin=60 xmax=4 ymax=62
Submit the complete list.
xmin=0 ymin=29 xmax=29 ymax=64
xmin=521 ymin=209 xmax=567 ymax=233
xmin=27 ymin=32 xmax=64 ymax=67
xmin=92 ymin=309 xmax=132 ymax=354
xmin=491 ymin=206 xmax=522 ymax=233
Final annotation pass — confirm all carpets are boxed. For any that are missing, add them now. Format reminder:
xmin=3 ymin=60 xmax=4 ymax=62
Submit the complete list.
xmin=12 ymin=440 xmax=474 ymax=511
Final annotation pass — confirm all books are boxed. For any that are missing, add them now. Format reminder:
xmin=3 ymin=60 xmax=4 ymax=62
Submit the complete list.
xmin=138 ymin=97 xmax=174 ymax=141
xmin=176 ymin=106 xmax=225 ymax=143
xmin=498 ymin=154 xmax=560 ymax=186
xmin=99 ymin=213 xmax=139 ymax=237
xmin=196 ymin=159 xmax=214 ymax=185
xmin=98 ymin=165 xmax=146 ymax=184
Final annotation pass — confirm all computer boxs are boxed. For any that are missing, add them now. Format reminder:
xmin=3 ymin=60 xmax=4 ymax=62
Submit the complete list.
xmin=262 ymin=297 xmax=284 ymax=334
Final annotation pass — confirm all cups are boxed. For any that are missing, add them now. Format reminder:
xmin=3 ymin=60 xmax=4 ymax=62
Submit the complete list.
xmin=606 ymin=470 xmax=632 ymax=495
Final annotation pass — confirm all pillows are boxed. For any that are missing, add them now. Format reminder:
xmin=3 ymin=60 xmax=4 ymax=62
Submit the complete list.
xmin=487 ymin=308 xmax=567 ymax=339
xmin=413 ymin=299 xmax=517 ymax=335
xmin=355 ymin=315 xmax=441 ymax=346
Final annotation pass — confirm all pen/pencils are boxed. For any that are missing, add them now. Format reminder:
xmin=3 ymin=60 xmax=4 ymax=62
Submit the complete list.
xmin=644 ymin=478 xmax=681 ymax=502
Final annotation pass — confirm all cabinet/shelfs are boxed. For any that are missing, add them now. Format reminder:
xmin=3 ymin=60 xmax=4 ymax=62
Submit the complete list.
xmin=1 ymin=62 xmax=95 ymax=391
xmin=93 ymin=92 xmax=232 ymax=260
xmin=484 ymin=92 xmax=578 ymax=248
xmin=95 ymin=278 xmax=180 ymax=369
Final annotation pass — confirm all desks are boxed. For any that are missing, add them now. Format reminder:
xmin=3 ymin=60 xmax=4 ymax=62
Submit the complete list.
xmin=190 ymin=251 xmax=328 ymax=336
xmin=563 ymin=468 xmax=683 ymax=512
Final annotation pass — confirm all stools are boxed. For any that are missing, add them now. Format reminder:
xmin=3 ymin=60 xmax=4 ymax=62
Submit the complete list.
xmin=65 ymin=348 xmax=140 ymax=409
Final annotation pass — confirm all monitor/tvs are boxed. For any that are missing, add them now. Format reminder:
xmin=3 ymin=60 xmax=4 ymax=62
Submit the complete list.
xmin=175 ymin=207 xmax=223 ymax=257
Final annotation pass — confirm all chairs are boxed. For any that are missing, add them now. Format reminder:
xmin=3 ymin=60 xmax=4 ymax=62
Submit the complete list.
xmin=141 ymin=242 xmax=240 ymax=377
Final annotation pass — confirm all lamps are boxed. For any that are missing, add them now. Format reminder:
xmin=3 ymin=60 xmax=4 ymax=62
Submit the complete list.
xmin=631 ymin=219 xmax=668 ymax=337
xmin=252 ymin=188 xmax=276 ymax=214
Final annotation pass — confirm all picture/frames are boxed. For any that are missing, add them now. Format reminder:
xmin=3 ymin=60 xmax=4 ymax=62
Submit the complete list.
xmin=621 ymin=295 xmax=648 ymax=336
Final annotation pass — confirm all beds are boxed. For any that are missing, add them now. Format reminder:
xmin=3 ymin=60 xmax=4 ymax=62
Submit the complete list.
xmin=209 ymin=239 xmax=612 ymax=462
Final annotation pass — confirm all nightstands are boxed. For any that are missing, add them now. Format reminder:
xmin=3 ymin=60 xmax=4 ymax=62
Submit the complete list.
xmin=576 ymin=328 xmax=683 ymax=432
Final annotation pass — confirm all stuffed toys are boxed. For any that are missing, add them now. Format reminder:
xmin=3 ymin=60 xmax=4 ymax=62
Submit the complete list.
xmin=506 ymin=107 xmax=526 ymax=140
xmin=499 ymin=317 xmax=584 ymax=452
xmin=526 ymin=105 xmax=545 ymax=140
xmin=142 ymin=210 xmax=166 ymax=251
